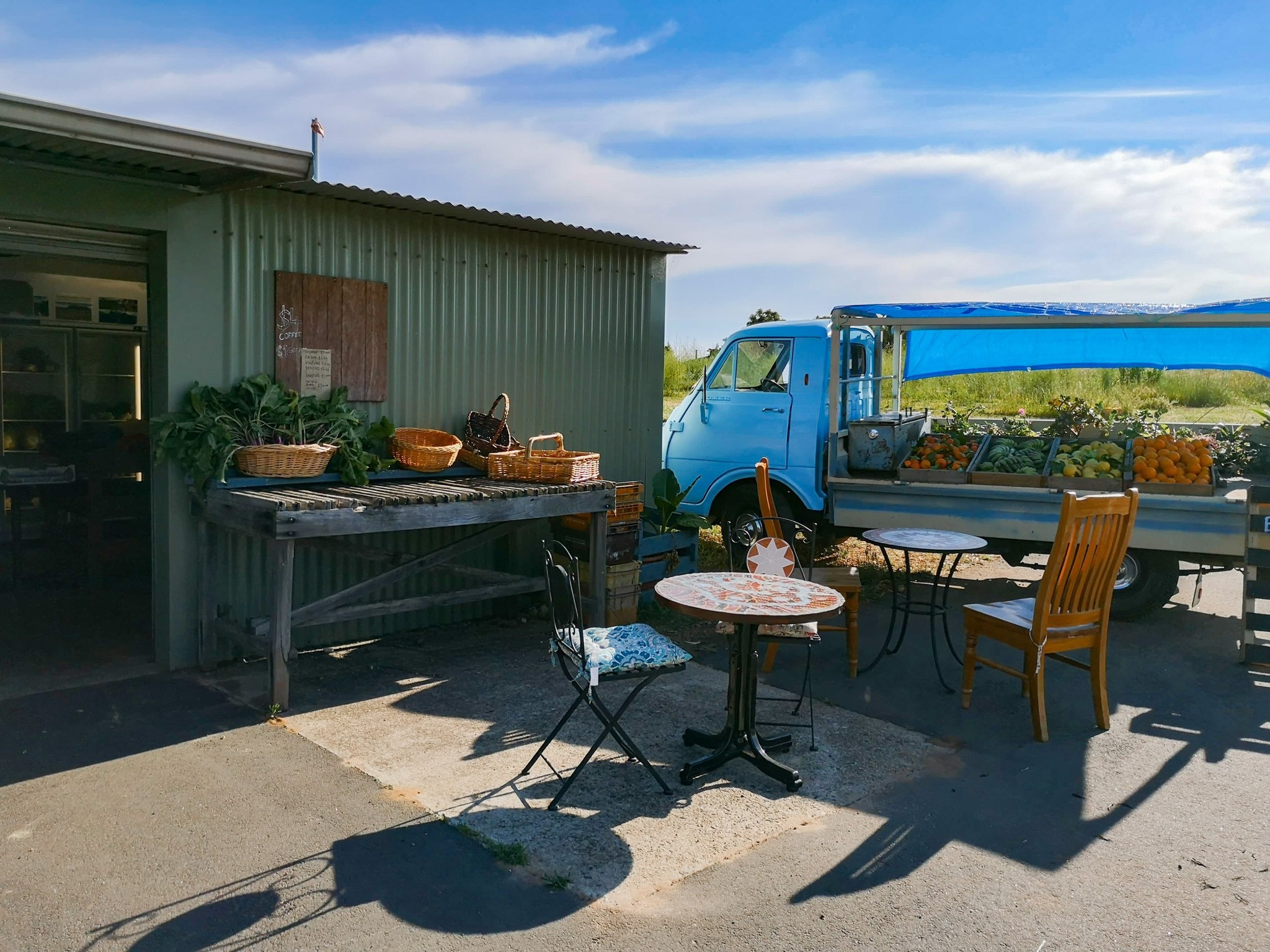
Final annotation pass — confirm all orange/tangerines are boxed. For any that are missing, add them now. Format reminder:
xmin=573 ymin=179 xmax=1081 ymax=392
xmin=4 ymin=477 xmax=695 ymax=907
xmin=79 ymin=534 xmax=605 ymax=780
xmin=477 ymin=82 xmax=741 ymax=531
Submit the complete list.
xmin=1132 ymin=434 xmax=1213 ymax=485
xmin=904 ymin=432 xmax=979 ymax=471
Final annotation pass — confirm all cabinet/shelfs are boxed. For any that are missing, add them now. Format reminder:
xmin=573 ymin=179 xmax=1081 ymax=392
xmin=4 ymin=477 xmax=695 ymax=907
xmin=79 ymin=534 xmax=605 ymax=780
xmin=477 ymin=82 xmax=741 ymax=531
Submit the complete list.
xmin=1 ymin=327 xmax=75 ymax=518
xmin=76 ymin=326 xmax=144 ymax=525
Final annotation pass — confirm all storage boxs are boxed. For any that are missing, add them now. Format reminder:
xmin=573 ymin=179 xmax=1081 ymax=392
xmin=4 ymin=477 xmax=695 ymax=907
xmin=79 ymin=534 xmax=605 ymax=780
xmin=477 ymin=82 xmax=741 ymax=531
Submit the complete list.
xmin=1126 ymin=439 xmax=1217 ymax=497
xmin=1044 ymin=437 xmax=1132 ymax=494
xmin=897 ymin=432 xmax=989 ymax=484
xmin=970 ymin=434 xmax=1057 ymax=490
xmin=548 ymin=478 xmax=644 ymax=629
xmin=849 ymin=411 xmax=932 ymax=471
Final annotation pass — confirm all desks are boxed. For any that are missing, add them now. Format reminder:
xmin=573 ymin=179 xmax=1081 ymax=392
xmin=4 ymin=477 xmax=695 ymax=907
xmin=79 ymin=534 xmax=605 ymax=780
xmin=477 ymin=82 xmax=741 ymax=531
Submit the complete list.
xmin=191 ymin=464 xmax=619 ymax=714
xmin=655 ymin=571 xmax=848 ymax=792
xmin=857 ymin=527 xmax=988 ymax=694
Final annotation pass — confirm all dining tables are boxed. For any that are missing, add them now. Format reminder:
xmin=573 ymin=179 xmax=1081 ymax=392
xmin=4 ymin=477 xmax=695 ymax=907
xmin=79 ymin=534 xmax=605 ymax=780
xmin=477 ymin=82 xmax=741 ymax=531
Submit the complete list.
xmin=1 ymin=475 xmax=92 ymax=593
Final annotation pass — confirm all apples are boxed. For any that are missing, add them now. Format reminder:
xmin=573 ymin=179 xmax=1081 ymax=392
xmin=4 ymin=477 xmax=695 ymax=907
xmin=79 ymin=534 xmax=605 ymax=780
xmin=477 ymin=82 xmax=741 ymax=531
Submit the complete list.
xmin=1050 ymin=440 xmax=1126 ymax=478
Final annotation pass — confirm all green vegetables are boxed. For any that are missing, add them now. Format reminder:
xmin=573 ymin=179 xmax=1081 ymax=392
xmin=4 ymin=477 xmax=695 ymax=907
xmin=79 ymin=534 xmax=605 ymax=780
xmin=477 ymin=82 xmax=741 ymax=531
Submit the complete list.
xmin=148 ymin=373 xmax=399 ymax=517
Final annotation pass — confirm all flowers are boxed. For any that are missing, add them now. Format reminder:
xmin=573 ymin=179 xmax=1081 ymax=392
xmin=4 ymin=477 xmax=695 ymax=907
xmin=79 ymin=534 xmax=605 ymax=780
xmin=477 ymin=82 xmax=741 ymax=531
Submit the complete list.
xmin=1013 ymin=408 xmax=1025 ymax=426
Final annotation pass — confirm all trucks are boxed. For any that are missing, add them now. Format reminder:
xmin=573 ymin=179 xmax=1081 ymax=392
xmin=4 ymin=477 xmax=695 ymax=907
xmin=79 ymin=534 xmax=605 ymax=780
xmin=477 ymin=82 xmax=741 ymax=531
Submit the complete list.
xmin=662 ymin=296 xmax=1270 ymax=623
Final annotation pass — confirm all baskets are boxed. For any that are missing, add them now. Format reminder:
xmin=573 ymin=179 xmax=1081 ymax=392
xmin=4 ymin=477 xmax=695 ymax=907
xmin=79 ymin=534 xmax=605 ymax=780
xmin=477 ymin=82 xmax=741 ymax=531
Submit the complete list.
xmin=455 ymin=437 xmax=526 ymax=471
xmin=487 ymin=433 xmax=599 ymax=483
xmin=389 ymin=427 xmax=462 ymax=471
xmin=231 ymin=442 xmax=340 ymax=476
xmin=464 ymin=393 xmax=512 ymax=453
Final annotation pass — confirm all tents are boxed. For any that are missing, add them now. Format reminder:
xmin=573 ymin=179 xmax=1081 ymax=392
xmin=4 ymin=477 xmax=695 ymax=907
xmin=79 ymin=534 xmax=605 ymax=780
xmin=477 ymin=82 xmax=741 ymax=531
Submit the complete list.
xmin=830 ymin=293 xmax=1270 ymax=383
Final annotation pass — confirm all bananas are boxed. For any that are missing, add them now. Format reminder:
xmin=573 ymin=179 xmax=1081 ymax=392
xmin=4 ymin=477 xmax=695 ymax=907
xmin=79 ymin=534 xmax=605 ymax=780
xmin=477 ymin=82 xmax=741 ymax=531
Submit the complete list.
xmin=977 ymin=438 xmax=1051 ymax=475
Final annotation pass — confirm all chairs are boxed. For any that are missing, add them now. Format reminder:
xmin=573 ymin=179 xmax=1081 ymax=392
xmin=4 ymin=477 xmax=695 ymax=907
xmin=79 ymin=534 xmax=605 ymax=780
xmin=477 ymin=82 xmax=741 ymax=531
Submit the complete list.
xmin=756 ymin=456 xmax=862 ymax=681
xmin=522 ymin=539 xmax=693 ymax=812
xmin=962 ymin=487 xmax=1140 ymax=744
xmin=726 ymin=518 xmax=824 ymax=752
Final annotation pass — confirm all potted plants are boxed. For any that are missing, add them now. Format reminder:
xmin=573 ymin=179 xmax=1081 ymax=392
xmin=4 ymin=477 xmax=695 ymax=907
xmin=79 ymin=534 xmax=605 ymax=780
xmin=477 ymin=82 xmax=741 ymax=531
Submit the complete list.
xmin=638 ymin=467 xmax=714 ymax=591
xmin=1211 ymin=422 xmax=1258 ymax=466
